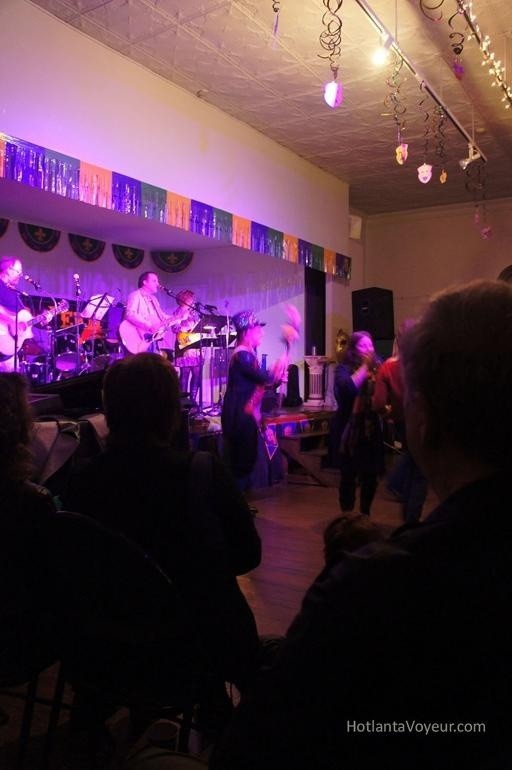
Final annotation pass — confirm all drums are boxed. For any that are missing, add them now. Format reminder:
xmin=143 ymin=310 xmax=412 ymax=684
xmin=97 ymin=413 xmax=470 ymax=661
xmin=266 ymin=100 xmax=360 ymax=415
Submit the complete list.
xmin=54 ymin=333 xmax=79 ymax=370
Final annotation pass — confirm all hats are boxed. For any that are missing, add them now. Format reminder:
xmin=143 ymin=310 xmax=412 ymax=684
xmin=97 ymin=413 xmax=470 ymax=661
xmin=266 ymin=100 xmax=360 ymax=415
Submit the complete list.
xmin=230 ymin=310 xmax=267 ymax=331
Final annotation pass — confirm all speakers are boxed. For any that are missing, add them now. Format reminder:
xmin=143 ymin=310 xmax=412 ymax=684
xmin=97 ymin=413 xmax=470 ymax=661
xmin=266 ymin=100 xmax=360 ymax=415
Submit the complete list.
xmin=352 ymin=287 xmax=395 ymax=340
xmin=349 ymin=214 xmax=362 ymax=240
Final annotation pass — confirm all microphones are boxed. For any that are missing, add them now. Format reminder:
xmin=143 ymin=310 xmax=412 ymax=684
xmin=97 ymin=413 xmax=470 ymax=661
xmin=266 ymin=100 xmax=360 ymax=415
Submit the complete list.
xmin=25 ymin=276 xmax=40 ymax=290
xmin=7 ymin=285 xmax=28 ymax=296
xmin=159 ymin=284 xmax=172 ymax=294
xmin=72 ymin=271 xmax=81 ymax=297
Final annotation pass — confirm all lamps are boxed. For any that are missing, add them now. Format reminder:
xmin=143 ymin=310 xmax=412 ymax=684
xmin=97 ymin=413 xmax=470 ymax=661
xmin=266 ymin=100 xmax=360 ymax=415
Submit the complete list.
xmin=458 ymin=140 xmax=482 ymax=171
xmin=371 ymin=31 xmax=396 ymax=69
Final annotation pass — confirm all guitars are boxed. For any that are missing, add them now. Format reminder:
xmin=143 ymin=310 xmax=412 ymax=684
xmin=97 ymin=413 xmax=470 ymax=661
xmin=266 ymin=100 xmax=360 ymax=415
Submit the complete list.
xmin=0 ymin=300 xmax=70 ymax=356
xmin=118 ymin=309 xmax=188 ymax=355
